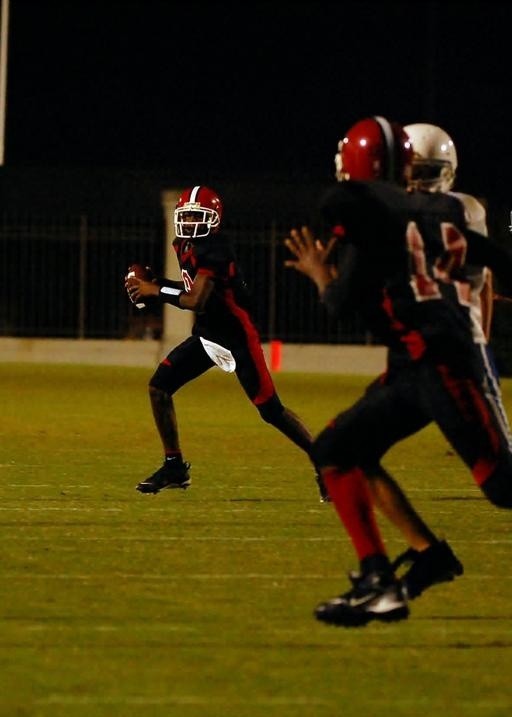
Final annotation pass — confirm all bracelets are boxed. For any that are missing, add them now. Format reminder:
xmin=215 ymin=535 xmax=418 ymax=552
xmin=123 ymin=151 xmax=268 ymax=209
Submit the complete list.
xmin=160 ymin=280 xmax=187 ymax=311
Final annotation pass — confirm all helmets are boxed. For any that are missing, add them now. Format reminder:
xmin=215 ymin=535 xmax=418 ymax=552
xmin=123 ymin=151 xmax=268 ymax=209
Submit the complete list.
xmin=335 ymin=116 xmax=458 ymax=194
xmin=174 ymin=186 xmax=223 ymax=238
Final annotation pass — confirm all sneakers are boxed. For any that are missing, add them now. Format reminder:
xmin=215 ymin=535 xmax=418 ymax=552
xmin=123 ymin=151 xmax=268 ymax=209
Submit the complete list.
xmin=313 ymin=539 xmax=464 ymax=628
xmin=136 ymin=461 xmax=191 ymax=494
xmin=317 ymin=476 xmax=334 ymax=503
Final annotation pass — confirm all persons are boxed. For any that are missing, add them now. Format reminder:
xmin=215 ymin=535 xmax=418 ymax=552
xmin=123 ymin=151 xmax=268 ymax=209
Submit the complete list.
xmin=279 ymin=116 xmax=510 ymax=628
xmin=367 ymin=118 xmax=495 ymax=603
xmin=127 ymin=181 xmax=337 ymax=506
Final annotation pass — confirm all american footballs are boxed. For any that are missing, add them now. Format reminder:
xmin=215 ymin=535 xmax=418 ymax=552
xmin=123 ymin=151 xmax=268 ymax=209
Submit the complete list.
xmin=124 ymin=261 xmax=154 ymax=311
